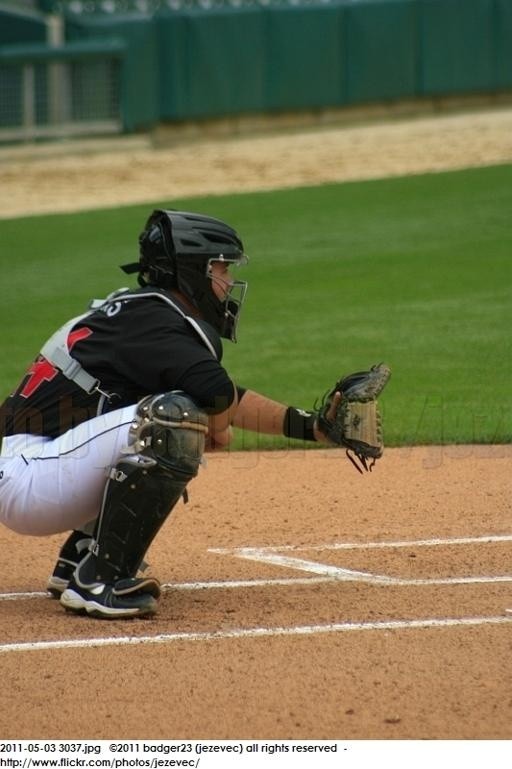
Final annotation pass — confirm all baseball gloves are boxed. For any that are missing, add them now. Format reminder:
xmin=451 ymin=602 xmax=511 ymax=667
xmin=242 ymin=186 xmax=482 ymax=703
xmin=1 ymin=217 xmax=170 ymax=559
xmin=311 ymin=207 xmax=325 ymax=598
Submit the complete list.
xmin=316 ymin=363 xmax=392 ymax=473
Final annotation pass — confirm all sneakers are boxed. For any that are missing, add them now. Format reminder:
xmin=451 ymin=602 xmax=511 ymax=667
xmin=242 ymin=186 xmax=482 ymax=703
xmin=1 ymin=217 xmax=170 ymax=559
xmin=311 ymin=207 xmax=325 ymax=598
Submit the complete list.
xmin=48 ymin=553 xmax=157 ymax=618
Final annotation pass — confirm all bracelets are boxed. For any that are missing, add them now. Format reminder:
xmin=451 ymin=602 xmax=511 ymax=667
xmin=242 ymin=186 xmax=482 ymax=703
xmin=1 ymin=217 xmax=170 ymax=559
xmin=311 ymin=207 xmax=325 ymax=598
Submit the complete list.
xmin=283 ymin=406 xmax=319 ymax=443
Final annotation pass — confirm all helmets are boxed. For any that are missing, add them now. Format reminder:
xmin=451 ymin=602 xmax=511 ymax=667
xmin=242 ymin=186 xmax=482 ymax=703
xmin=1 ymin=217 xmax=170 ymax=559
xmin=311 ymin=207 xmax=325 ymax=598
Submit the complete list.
xmin=139 ymin=209 xmax=247 ymax=344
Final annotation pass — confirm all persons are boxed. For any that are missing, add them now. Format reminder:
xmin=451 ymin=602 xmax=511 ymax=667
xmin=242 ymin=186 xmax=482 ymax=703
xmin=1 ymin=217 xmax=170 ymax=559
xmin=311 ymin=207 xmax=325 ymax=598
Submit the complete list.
xmin=0 ymin=208 xmax=392 ymax=620
